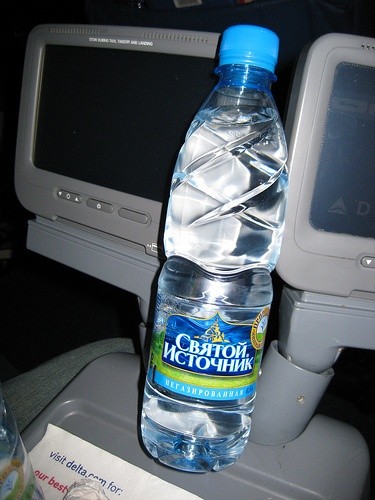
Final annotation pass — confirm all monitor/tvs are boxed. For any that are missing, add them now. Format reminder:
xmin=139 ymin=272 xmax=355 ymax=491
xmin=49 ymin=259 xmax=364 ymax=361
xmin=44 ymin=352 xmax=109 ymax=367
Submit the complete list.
xmin=14 ymin=25 xmax=221 ymax=260
xmin=274 ymin=33 xmax=375 ymax=298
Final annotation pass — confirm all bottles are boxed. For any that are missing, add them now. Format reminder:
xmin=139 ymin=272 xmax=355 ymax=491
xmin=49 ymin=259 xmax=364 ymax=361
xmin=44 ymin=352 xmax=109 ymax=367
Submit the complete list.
xmin=0 ymin=397 xmax=45 ymax=500
xmin=139 ymin=25 xmax=290 ymax=474
